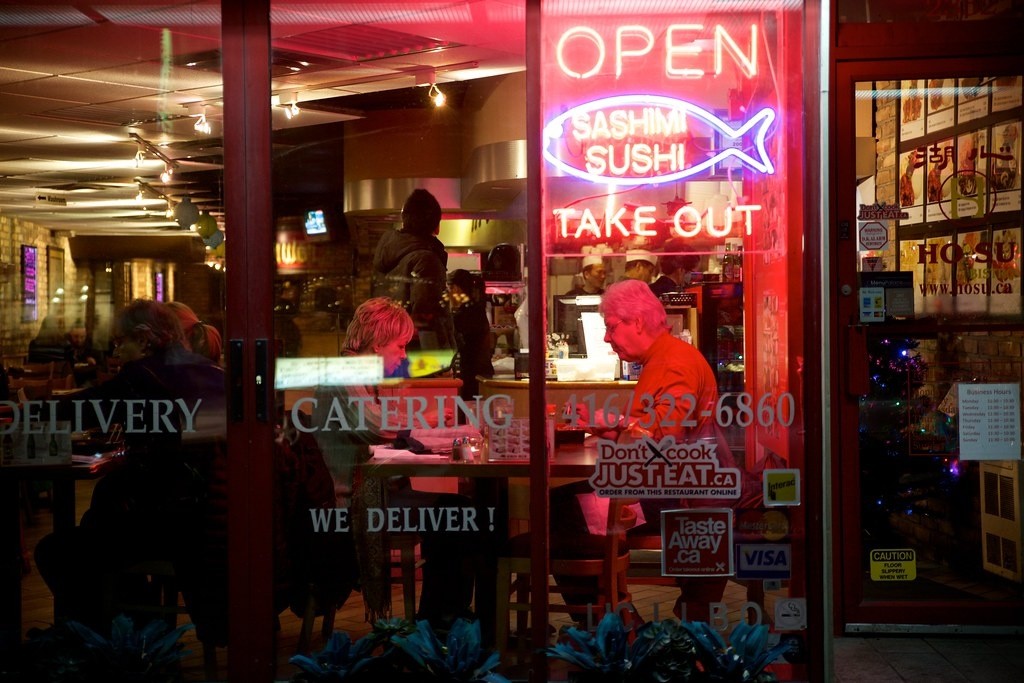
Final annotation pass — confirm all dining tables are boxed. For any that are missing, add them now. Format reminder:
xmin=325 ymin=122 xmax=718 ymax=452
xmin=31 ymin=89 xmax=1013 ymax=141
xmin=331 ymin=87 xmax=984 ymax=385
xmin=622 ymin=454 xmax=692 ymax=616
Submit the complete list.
xmin=343 ymin=422 xmax=634 ymax=636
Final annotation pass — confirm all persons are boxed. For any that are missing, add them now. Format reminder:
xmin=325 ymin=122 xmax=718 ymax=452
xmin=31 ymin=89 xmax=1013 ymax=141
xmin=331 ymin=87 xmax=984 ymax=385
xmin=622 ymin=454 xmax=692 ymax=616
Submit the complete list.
xmin=643 ymin=242 xmax=701 ymax=307
xmin=62 ymin=317 xmax=98 ymax=386
xmin=308 ymin=296 xmax=486 ymax=633
xmin=81 ymin=302 xmax=224 ymax=562
xmin=2 ymin=296 xmax=231 ymax=649
xmin=564 ymin=255 xmax=609 ymax=299
xmin=444 ymin=266 xmax=496 ymax=426
xmin=25 ymin=315 xmax=81 ymax=389
xmin=535 ymin=278 xmax=725 ymax=634
xmin=372 ymin=187 xmax=461 ymax=381
xmin=610 ymin=249 xmax=658 ymax=294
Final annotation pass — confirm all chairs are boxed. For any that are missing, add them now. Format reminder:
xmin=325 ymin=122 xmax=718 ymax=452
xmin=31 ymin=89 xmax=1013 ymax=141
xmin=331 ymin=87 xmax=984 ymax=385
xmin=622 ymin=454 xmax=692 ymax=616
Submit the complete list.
xmin=287 ymin=408 xmax=434 ymax=657
xmin=494 ymin=441 xmax=635 ymax=660
